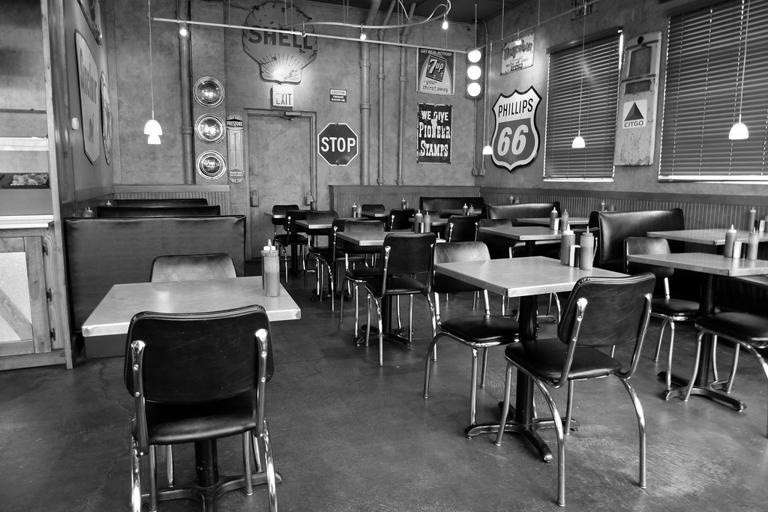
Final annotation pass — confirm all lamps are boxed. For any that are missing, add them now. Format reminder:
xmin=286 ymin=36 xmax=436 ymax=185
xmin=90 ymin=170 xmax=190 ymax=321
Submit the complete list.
xmin=143 ymin=1 xmax=164 ymax=147
xmin=571 ymin=1 xmax=588 ymax=150
xmin=728 ymin=0 xmax=752 ymax=142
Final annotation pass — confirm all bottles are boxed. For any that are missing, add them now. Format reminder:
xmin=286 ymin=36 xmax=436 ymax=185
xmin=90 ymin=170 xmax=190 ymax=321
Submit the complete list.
xmin=578 ymin=225 xmax=596 ymax=271
xmin=725 ymin=205 xmax=768 ymax=260
xmin=600 ymin=199 xmax=606 ymax=212
xmin=105 ymin=200 xmax=112 ymax=207
xmin=559 ymin=223 xmax=576 ymax=266
xmin=401 ymin=197 xmax=409 ymax=211
xmin=463 ymin=203 xmax=475 ymax=216
xmin=413 ymin=209 xmax=432 ymax=235
xmin=260 ymin=237 xmax=280 ymax=297
xmin=550 ymin=206 xmax=571 ymax=232
xmin=509 ymin=195 xmax=520 ymax=205
xmin=352 ymin=202 xmax=362 ymax=219
